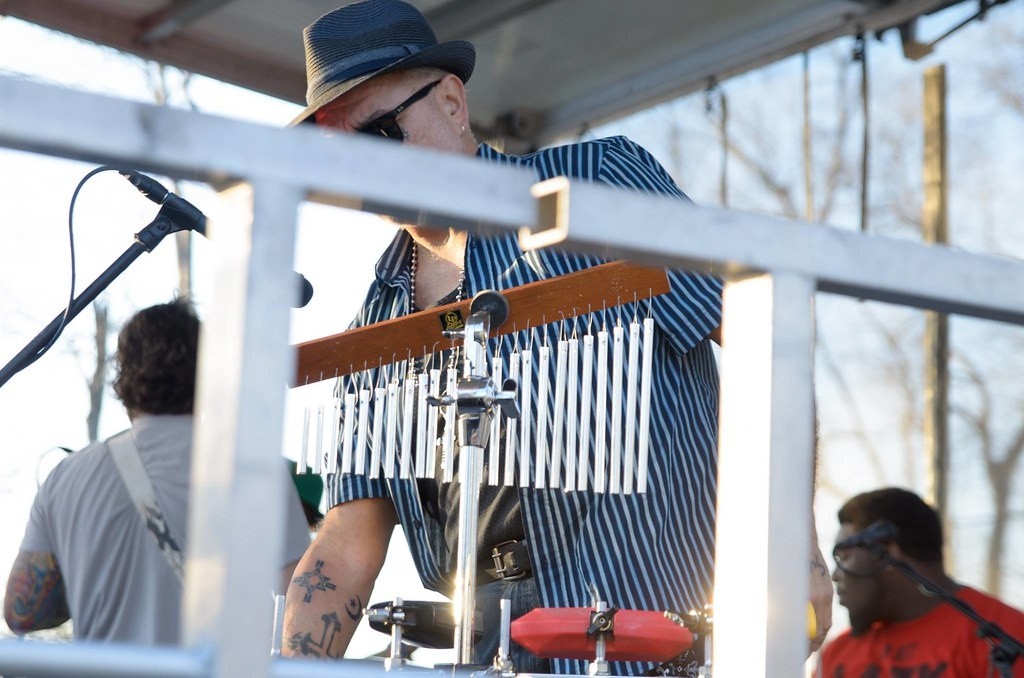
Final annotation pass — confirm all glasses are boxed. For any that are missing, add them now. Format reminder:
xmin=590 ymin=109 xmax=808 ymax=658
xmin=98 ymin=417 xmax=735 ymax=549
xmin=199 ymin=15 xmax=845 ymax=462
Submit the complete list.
xmin=356 ymin=78 xmax=441 ymax=144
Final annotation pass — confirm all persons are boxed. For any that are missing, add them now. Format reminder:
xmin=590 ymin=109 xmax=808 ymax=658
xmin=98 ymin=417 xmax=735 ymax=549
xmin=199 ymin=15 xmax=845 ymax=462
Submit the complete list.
xmin=3 ymin=305 xmax=316 ymax=646
xmin=808 ymin=488 xmax=1024 ymax=676
xmin=279 ymin=0 xmax=833 ymax=678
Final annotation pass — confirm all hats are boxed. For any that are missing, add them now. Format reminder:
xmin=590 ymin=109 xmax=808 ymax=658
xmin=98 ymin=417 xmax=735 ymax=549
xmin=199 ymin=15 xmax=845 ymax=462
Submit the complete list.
xmin=288 ymin=1 xmax=476 ymax=129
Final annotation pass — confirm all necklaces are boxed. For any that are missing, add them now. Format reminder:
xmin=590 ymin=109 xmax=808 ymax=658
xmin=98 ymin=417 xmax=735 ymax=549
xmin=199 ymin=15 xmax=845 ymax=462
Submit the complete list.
xmin=410 ymin=238 xmax=467 ymax=387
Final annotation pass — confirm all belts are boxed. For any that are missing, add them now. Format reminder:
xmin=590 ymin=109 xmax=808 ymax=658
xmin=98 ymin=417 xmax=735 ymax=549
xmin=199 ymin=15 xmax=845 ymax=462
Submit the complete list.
xmin=444 ymin=537 xmax=537 ymax=593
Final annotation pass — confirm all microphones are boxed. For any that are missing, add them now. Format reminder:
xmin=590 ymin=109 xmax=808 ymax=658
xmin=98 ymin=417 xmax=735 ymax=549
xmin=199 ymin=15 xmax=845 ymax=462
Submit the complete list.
xmin=119 ymin=170 xmax=313 ymax=309
xmin=840 ymin=519 xmax=899 ymax=553
